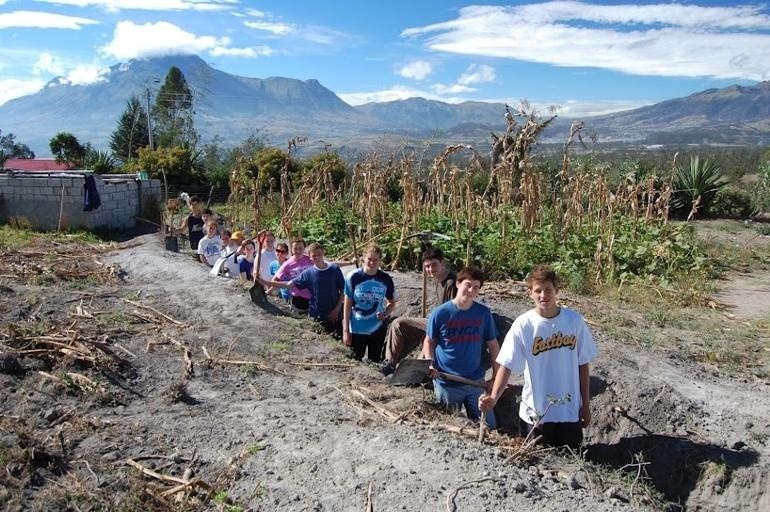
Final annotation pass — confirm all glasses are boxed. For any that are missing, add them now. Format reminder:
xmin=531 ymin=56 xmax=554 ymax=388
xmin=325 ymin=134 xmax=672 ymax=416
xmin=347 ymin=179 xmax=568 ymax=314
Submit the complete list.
xmin=276 ymin=248 xmax=287 ymax=254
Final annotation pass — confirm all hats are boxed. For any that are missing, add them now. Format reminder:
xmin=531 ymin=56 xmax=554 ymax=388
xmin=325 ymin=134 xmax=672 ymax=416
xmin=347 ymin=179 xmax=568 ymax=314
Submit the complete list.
xmin=230 ymin=232 xmax=243 ymax=240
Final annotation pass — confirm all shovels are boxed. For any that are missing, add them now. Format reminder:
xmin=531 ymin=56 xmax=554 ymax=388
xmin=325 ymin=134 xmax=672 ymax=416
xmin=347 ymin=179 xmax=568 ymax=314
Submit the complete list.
xmin=165 ymin=199 xmax=178 ymax=253
xmin=389 ymin=358 xmax=488 ymax=389
xmin=211 ymin=236 xmax=257 ymax=276
xmin=249 ymin=229 xmax=267 ymax=303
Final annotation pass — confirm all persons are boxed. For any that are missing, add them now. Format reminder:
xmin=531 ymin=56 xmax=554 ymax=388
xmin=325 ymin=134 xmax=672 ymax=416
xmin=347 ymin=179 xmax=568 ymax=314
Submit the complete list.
xmin=240 ymin=240 xmax=256 ymax=282
xmin=253 ymin=231 xmax=277 ymax=291
xmin=220 ymin=230 xmax=232 ymax=274
xmin=267 ymin=243 xmax=291 ymax=305
xmin=478 ymin=266 xmax=598 ymax=452
xmin=425 ymin=267 xmax=500 ymax=431
xmin=226 ymin=232 xmax=247 ymax=277
xmin=201 ymin=209 xmax=212 ymax=236
xmin=342 ymin=246 xmax=398 ymax=362
xmin=265 ymin=237 xmax=314 ymax=316
xmin=379 ymin=246 xmax=458 ymax=376
xmin=253 ymin=243 xmax=345 ymax=333
xmin=168 ymin=196 xmax=205 ymax=258
xmin=198 ymin=221 xmax=222 ymax=268
